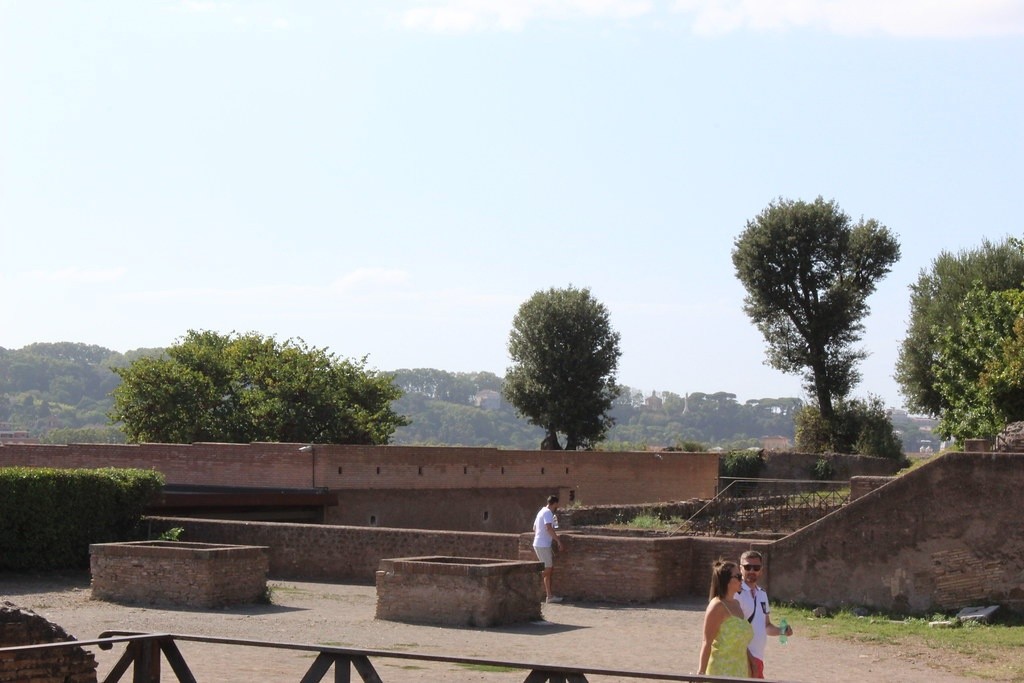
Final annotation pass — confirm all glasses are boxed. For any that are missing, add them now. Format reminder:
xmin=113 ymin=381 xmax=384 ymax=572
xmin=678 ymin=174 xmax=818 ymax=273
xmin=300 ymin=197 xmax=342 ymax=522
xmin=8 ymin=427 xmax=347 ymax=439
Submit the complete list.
xmin=731 ymin=574 xmax=742 ymax=582
xmin=741 ymin=564 xmax=761 ymax=571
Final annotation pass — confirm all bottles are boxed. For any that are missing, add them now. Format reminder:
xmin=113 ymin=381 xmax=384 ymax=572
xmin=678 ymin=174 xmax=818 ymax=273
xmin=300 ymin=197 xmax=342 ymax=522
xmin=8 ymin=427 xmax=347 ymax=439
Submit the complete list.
xmin=553 ymin=515 xmax=558 ymax=528
xmin=779 ymin=619 xmax=788 ymax=644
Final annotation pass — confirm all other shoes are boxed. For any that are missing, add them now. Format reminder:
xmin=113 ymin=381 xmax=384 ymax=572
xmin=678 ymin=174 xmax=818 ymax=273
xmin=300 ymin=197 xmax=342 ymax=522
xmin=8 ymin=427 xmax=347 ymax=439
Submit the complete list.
xmin=546 ymin=595 xmax=563 ymax=603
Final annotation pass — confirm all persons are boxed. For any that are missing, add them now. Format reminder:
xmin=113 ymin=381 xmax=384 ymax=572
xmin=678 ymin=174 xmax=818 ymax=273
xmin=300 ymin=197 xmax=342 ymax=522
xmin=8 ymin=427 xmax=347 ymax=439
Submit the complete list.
xmin=698 ymin=555 xmax=758 ymax=679
xmin=733 ymin=550 xmax=793 ymax=679
xmin=532 ymin=495 xmax=564 ymax=603
